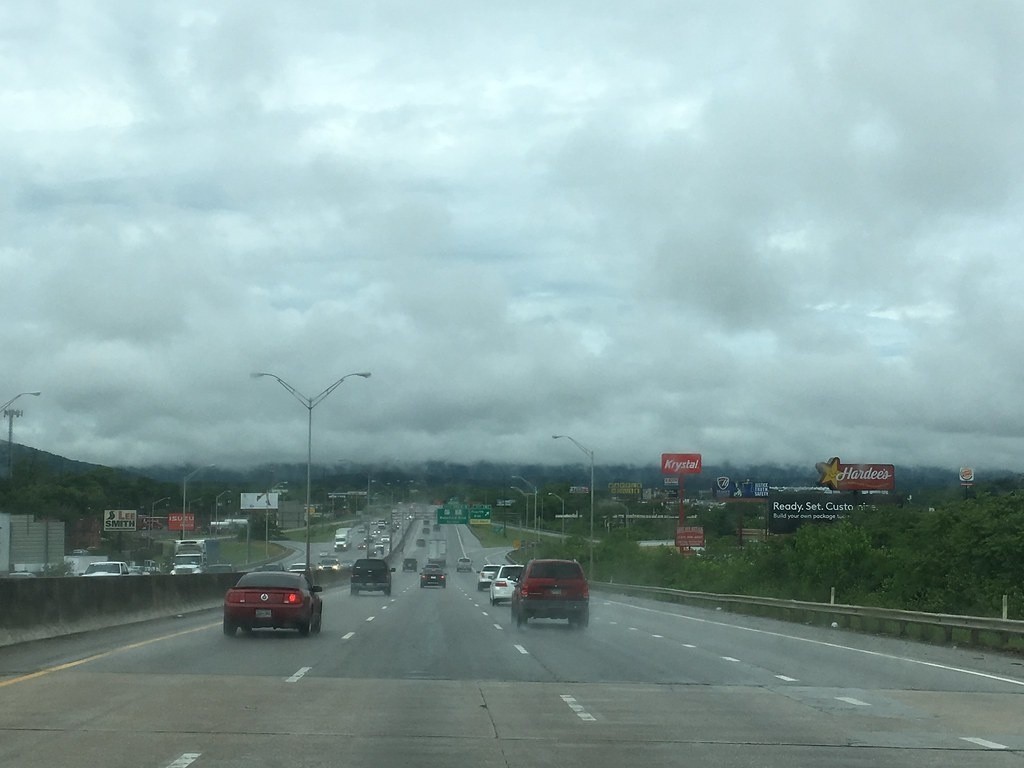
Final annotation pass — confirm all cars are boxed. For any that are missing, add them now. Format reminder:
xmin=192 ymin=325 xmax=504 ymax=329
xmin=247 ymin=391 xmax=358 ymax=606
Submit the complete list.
xmin=8 ymin=559 xmax=161 ymax=577
xmin=222 ymin=570 xmax=323 ymax=636
xmin=206 ymin=558 xmax=355 ymax=574
xmin=356 ymin=509 xmax=474 ymax=588
xmin=488 ymin=563 xmax=525 ymax=607
xmin=475 ymin=563 xmax=502 ymax=591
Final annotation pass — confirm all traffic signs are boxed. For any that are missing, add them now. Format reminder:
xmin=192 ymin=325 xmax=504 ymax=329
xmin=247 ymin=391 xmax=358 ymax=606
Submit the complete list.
xmin=469 ymin=508 xmax=491 ymax=525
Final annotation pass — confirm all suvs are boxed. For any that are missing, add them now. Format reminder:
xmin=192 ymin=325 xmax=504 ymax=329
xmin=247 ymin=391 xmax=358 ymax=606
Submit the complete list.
xmin=350 ymin=558 xmax=396 ymax=597
xmin=507 ymin=558 xmax=590 ymax=630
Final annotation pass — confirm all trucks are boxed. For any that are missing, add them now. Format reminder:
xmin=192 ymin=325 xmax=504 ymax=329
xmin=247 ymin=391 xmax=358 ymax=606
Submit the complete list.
xmin=333 ymin=527 xmax=353 ymax=552
xmin=168 ymin=539 xmax=208 ymax=576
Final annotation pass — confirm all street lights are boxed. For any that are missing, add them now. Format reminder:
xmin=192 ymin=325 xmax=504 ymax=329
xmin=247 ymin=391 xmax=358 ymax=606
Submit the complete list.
xmin=335 ymin=456 xmax=400 ymax=559
xmin=510 ymin=475 xmax=537 ymax=559
xmin=187 ymin=498 xmax=202 ymax=536
xmin=215 ymin=491 xmax=231 ymax=539
xmin=265 ymin=481 xmax=287 ymax=557
xmin=553 ymin=435 xmax=594 ymax=582
xmin=182 ymin=463 xmax=216 ymax=540
xmin=151 ymin=496 xmax=171 ymax=538
xmin=511 ymin=486 xmax=528 ymax=557
xmin=372 ymin=479 xmax=416 ymax=554
xmin=548 ymin=493 xmax=565 ymax=545
xmin=248 ymin=369 xmax=374 ymax=583
